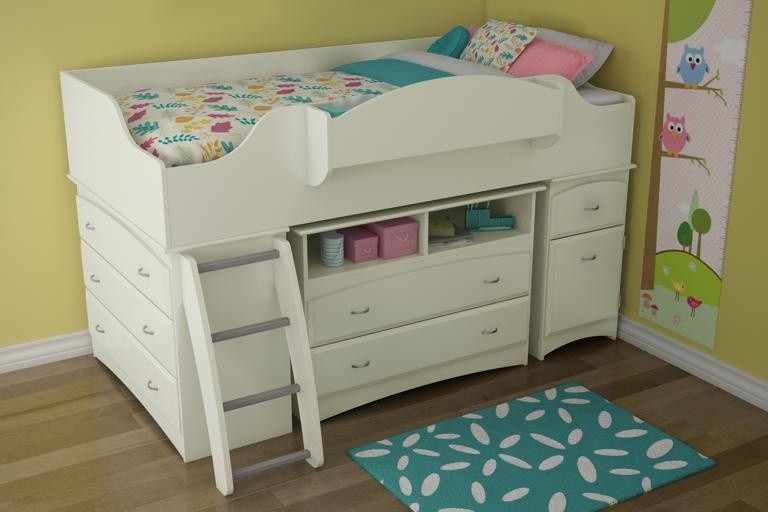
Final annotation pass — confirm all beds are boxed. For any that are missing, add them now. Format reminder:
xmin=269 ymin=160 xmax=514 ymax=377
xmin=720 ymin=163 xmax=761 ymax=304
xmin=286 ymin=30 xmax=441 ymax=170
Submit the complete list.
xmin=54 ymin=30 xmax=637 ymax=495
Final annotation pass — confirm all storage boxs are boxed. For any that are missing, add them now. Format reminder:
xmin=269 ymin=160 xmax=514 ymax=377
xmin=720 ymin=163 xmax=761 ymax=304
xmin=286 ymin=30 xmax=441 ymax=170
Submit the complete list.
xmin=362 ymin=214 xmax=420 ymax=261
xmin=340 ymin=227 xmax=379 ymax=263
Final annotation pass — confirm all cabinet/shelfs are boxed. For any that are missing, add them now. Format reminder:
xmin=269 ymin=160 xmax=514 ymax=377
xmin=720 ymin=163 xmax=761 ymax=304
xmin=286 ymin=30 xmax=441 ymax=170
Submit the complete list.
xmin=290 ymin=187 xmax=539 ymax=423
xmin=528 ymin=173 xmax=632 ymax=361
xmin=71 ymin=186 xmax=293 ymax=463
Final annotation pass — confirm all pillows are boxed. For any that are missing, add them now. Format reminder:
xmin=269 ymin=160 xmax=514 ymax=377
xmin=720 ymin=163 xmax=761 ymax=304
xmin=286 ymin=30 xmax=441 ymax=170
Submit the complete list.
xmin=463 ymin=23 xmax=481 ymax=41
xmin=427 ymin=26 xmax=468 ymax=63
xmin=461 ymin=18 xmax=541 ymax=74
xmin=537 ymin=29 xmax=617 ymax=93
xmin=507 ymin=39 xmax=596 ymax=86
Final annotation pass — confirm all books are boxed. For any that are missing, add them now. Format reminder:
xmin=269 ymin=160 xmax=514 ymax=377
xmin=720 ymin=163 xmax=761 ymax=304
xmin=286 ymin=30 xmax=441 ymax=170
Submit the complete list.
xmin=429 ymin=211 xmax=473 ymax=250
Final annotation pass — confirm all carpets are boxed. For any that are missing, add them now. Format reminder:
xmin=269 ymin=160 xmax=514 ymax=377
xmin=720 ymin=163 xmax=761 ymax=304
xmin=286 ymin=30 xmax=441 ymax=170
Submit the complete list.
xmin=344 ymin=379 xmax=717 ymax=511
